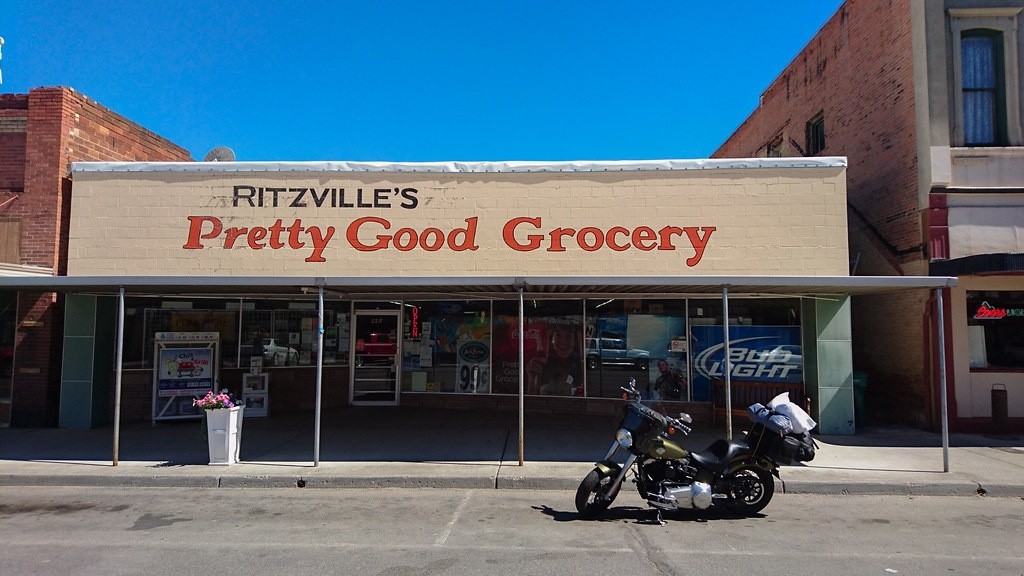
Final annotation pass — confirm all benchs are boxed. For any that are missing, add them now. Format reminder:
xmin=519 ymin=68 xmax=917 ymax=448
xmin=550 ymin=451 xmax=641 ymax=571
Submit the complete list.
xmin=711 ymin=381 xmax=811 ymax=427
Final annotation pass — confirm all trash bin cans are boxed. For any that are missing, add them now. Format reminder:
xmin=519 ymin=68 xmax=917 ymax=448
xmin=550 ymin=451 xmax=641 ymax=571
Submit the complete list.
xmin=990 ymin=383 xmax=1009 ymax=432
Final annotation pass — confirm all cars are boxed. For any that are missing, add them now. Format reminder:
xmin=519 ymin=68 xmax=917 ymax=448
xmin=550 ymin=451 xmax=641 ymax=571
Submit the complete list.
xmin=363 ymin=332 xmax=398 ymax=355
xmin=240 ymin=338 xmax=300 ymax=366
xmin=585 ymin=338 xmax=652 ymax=372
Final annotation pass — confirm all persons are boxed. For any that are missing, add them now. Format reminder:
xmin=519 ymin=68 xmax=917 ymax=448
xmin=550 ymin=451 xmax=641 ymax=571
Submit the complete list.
xmin=526 ymin=318 xmax=585 ymax=397
xmin=649 ymin=359 xmax=686 ymax=401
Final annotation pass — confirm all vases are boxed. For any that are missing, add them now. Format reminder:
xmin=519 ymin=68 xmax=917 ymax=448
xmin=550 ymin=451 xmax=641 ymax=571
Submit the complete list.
xmin=205 ymin=404 xmax=245 ymax=466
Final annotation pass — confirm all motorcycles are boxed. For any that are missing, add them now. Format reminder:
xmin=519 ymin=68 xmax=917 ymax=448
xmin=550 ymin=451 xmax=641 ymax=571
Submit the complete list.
xmin=572 ymin=377 xmax=819 ymax=519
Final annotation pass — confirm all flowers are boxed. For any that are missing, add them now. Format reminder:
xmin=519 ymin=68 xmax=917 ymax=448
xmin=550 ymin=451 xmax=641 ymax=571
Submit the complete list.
xmin=192 ymin=389 xmax=244 ymax=436
